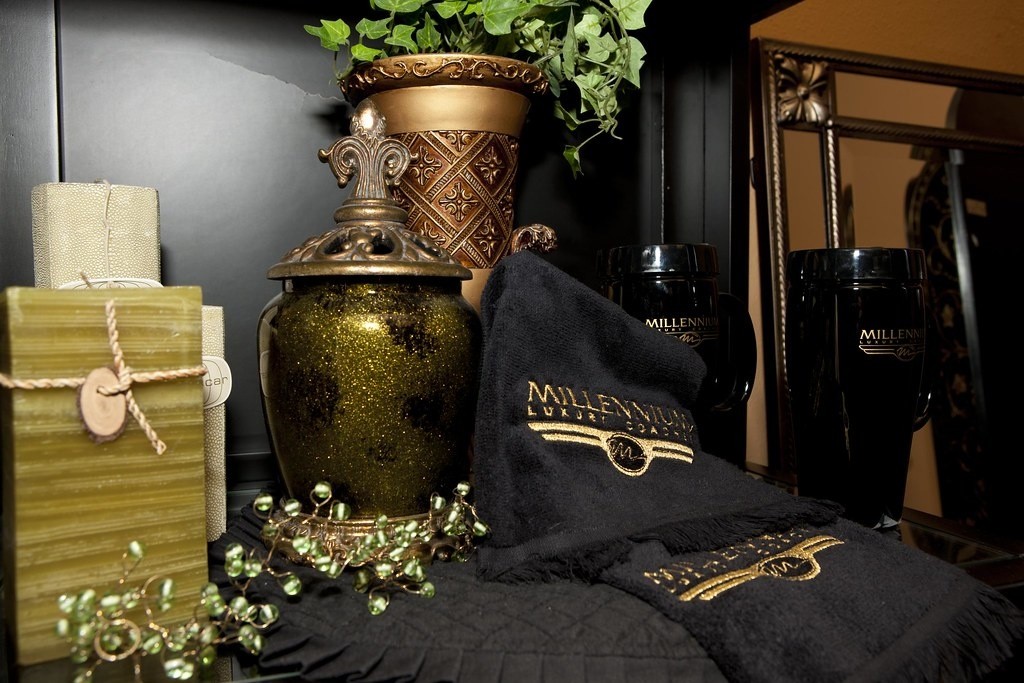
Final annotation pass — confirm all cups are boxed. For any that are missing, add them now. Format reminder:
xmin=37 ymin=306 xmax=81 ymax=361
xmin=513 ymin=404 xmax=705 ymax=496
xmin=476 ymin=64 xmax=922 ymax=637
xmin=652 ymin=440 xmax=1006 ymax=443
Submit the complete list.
xmin=783 ymin=245 xmax=941 ymax=537
xmin=581 ymin=243 xmax=758 ymax=470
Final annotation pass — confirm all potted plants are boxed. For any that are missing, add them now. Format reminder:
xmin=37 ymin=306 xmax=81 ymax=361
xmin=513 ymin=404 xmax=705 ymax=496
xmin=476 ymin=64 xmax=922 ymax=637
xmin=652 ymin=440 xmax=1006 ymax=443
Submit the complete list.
xmin=303 ymin=0 xmax=653 ymax=269
